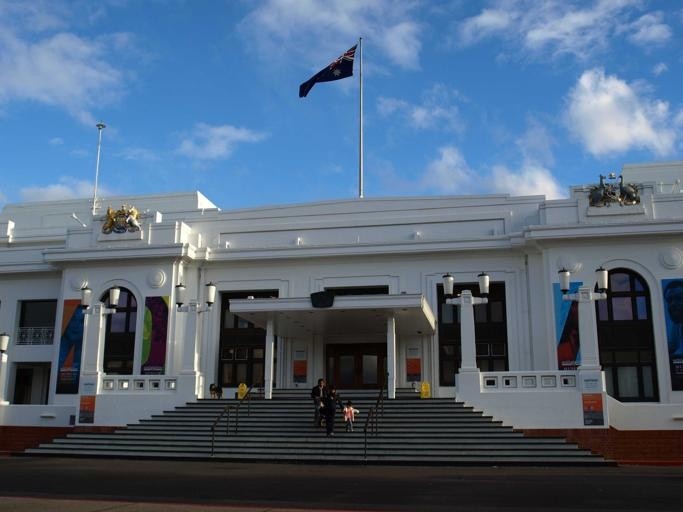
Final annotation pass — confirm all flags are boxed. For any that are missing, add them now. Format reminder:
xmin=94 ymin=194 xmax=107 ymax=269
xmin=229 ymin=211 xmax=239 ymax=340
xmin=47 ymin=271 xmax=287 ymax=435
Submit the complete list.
xmin=297 ymin=45 xmax=357 ymax=98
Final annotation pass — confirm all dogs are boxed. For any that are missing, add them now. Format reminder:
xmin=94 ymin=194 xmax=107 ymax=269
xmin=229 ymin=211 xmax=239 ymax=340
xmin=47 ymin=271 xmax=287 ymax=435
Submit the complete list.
xmin=210 ymin=383 xmax=223 ymax=400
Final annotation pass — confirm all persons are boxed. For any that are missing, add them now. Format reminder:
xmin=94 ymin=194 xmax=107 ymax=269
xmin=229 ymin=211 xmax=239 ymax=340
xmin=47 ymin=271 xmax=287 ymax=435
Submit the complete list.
xmin=324 ymin=384 xmax=344 ymax=436
xmin=310 ymin=377 xmax=325 ymax=425
xmin=342 ymin=399 xmax=359 ymax=432
